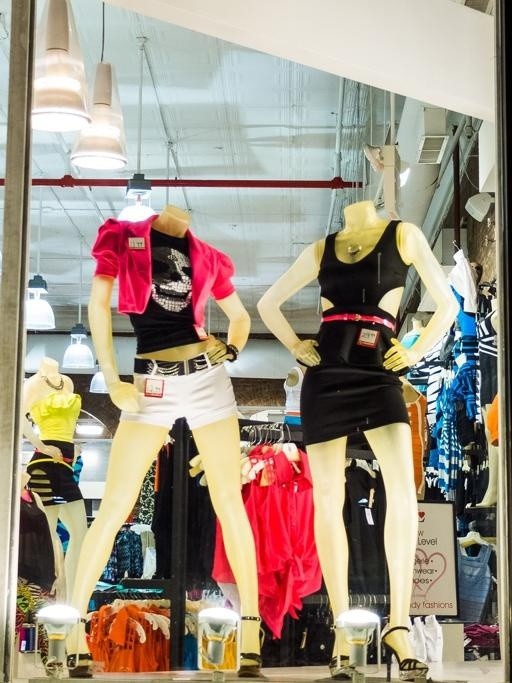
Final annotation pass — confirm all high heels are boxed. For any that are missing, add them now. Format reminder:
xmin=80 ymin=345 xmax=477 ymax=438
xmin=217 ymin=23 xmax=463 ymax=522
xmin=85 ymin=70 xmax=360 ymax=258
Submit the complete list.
xmin=65 ymin=616 xmax=95 ymax=681
xmin=325 ymin=621 xmax=358 ymax=680
xmin=237 ymin=613 xmax=263 ymax=679
xmin=380 ymin=623 xmax=431 ymax=682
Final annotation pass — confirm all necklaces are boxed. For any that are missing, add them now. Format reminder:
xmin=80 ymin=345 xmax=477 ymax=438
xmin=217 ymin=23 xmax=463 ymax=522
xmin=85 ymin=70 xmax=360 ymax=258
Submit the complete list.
xmin=38 ymin=373 xmax=64 ymax=390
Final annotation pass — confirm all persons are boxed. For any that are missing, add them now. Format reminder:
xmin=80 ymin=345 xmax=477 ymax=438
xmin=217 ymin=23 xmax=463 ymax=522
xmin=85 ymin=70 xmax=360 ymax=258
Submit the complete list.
xmin=20 ymin=356 xmax=88 ymax=609
xmin=61 ymin=202 xmax=263 ymax=681
xmin=256 ymin=200 xmax=460 ymax=680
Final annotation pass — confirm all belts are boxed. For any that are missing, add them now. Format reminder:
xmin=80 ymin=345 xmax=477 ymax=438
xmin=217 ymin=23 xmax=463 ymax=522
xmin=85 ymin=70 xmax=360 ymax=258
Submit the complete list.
xmin=318 ymin=314 xmax=396 ymax=331
xmin=132 ymin=354 xmax=210 ymax=380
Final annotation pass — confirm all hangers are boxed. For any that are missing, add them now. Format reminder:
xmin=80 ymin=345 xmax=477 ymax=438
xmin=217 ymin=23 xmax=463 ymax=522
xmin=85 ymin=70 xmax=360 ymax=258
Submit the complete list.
xmin=90 ymin=581 xmax=188 ymax=644
xmin=197 ymin=590 xmax=392 ymax=619
xmin=456 ymin=515 xmax=496 ymax=587
xmin=241 ymin=423 xmax=306 ymax=480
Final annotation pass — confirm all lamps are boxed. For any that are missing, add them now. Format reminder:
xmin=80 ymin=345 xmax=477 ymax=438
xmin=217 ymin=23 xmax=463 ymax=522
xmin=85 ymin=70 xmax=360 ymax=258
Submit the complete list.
xmin=200 ymin=609 xmax=237 ymax=665
xmin=36 ymin=606 xmax=78 ymax=663
xmin=72 ymin=2 xmax=127 ymax=170
xmin=25 ymin=189 xmax=56 ymax=331
xmin=87 ymin=362 xmax=108 ymax=396
xmin=27 ymin=0 xmax=89 ymax=134
xmin=60 ymin=237 xmax=96 ymax=370
xmin=116 ymin=44 xmax=161 ymax=222
xmin=340 ymin=609 xmax=378 ymax=666
